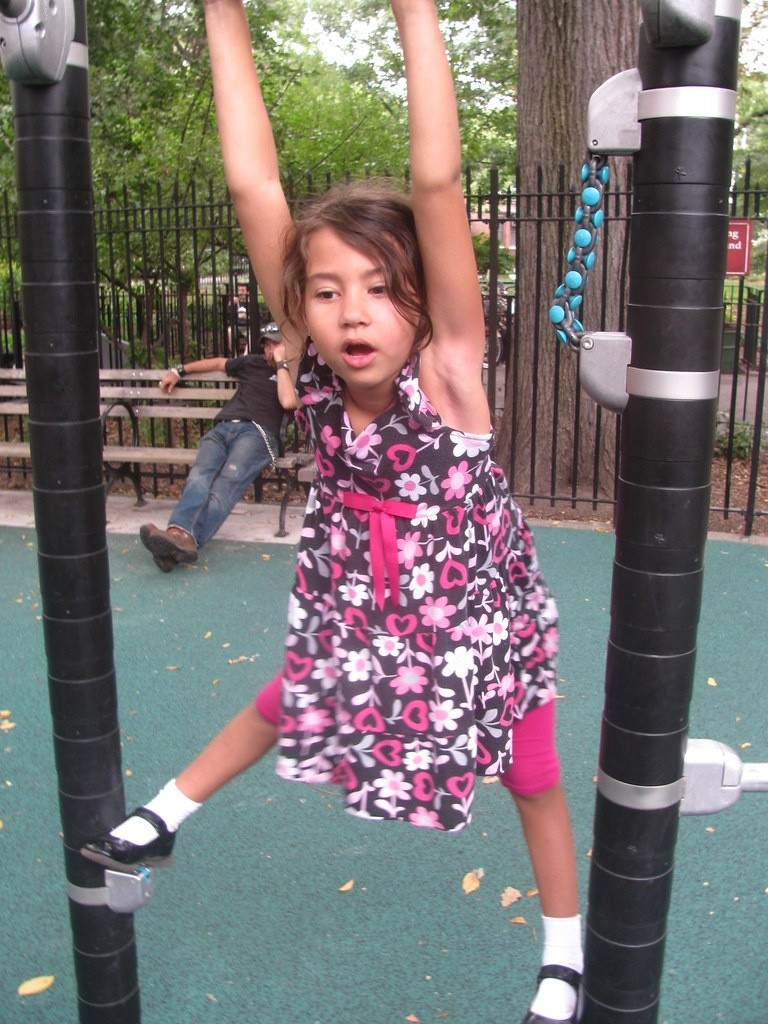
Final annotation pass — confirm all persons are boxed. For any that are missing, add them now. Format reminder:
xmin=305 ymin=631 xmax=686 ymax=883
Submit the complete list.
xmin=80 ymin=0 xmax=582 ymax=1024
xmin=138 ymin=323 xmax=297 ymax=573
xmin=226 ymin=283 xmax=252 ymax=357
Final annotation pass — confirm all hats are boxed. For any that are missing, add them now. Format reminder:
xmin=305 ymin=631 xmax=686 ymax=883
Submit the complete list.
xmin=257 ymin=322 xmax=283 ymax=345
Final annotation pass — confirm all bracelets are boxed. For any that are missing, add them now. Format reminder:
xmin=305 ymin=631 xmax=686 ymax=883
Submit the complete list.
xmin=172 ymin=363 xmax=187 ymax=378
xmin=276 ymin=360 xmax=290 ymax=371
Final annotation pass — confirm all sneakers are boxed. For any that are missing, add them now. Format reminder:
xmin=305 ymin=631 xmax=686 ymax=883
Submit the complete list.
xmin=140 ymin=524 xmax=198 ymax=573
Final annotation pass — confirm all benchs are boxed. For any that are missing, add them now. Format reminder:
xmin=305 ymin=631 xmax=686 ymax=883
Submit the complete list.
xmin=0 ymin=367 xmax=321 ymax=538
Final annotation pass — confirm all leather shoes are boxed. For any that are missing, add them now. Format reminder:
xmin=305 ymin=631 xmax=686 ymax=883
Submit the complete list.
xmin=80 ymin=807 xmax=179 ymax=871
xmin=523 ymin=964 xmax=582 ymax=1024
